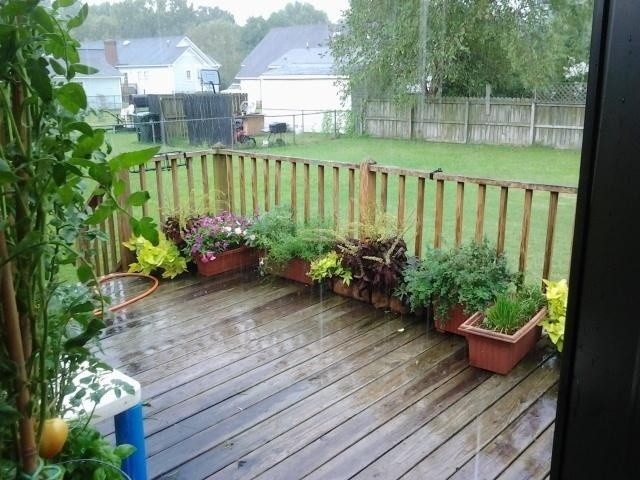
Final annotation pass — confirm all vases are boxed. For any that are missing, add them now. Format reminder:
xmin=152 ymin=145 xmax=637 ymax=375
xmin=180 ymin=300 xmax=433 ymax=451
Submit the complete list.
xmin=196 ymin=246 xmax=256 ymax=277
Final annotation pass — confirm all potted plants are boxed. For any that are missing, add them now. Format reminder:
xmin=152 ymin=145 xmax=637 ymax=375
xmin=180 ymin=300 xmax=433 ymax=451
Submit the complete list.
xmin=370 ymin=233 xmax=421 ymax=315
xmin=400 ymin=235 xmax=520 ymax=338
xmin=257 ymin=213 xmax=335 ymax=285
xmin=324 ymin=239 xmax=370 ymax=302
xmin=458 ymin=286 xmax=546 ymax=373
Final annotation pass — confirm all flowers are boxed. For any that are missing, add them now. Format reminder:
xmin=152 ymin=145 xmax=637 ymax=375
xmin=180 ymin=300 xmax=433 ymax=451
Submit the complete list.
xmin=181 ymin=212 xmax=256 ymax=264
